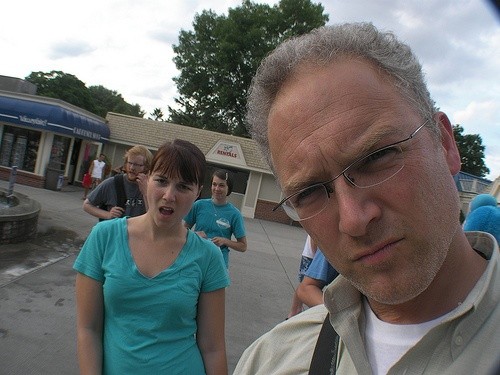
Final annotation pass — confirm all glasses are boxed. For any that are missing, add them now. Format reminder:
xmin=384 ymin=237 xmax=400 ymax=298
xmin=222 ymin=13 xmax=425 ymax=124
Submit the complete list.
xmin=126 ymin=160 xmax=146 ymax=168
xmin=271 ymin=119 xmax=433 ymax=222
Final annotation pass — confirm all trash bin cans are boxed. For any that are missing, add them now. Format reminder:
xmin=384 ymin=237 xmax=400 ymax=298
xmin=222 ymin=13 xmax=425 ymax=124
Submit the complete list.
xmin=45 ymin=169 xmax=64 ymax=191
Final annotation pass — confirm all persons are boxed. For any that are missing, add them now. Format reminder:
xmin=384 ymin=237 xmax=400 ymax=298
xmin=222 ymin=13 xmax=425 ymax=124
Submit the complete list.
xmin=297 ymin=250 xmax=339 ymax=307
xmin=82 ymin=145 xmax=154 ymax=222
xmin=75 ymin=138 xmax=231 ymax=375
xmin=80 ymin=153 xmax=106 ymax=200
xmin=232 ymin=23 xmax=500 ymax=375
xmin=285 ymin=234 xmax=318 ymax=319
xmin=184 ymin=168 xmax=249 ymax=255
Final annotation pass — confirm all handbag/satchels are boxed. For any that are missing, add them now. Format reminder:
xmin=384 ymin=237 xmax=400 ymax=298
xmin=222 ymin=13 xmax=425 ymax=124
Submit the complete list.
xmin=82 ymin=174 xmax=91 ymax=188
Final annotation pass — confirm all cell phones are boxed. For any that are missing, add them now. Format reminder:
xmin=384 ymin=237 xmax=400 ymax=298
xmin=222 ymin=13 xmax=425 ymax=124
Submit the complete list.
xmin=135 ymin=168 xmax=149 ymax=182
